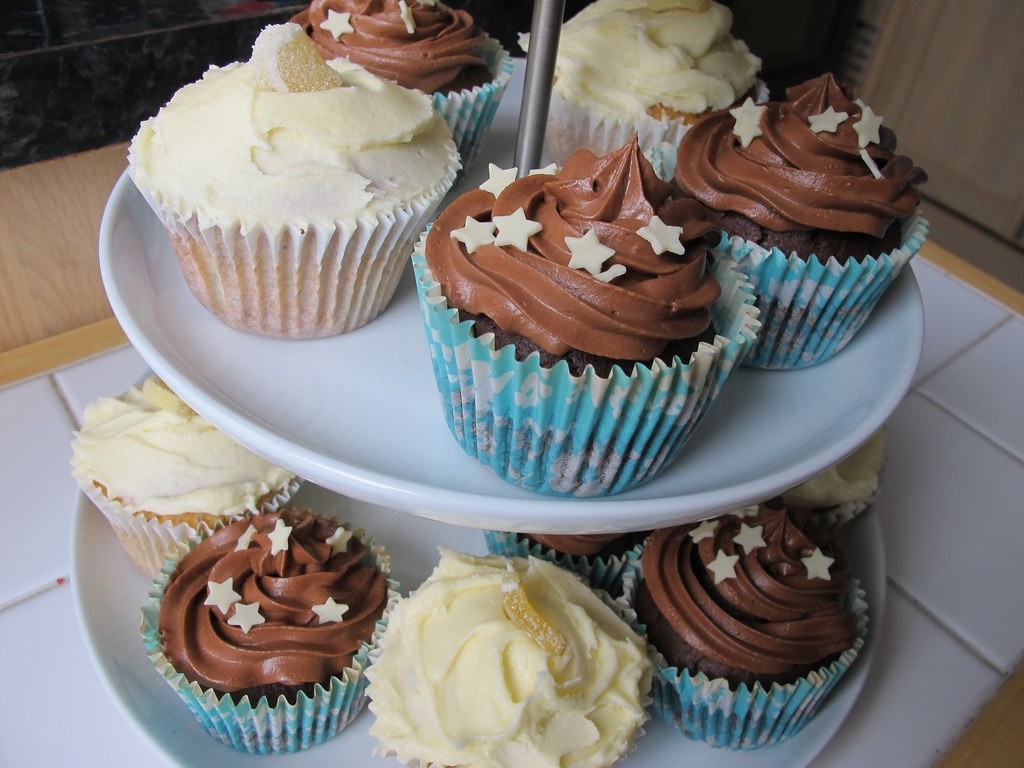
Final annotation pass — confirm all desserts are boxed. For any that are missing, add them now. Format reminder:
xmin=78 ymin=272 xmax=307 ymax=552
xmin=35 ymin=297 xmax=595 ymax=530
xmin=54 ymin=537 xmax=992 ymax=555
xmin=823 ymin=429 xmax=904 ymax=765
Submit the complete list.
xmin=126 ymin=0 xmax=930 ymax=500
xmin=68 ymin=370 xmax=888 ymax=768
